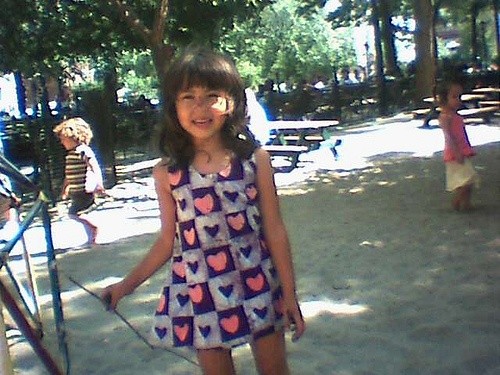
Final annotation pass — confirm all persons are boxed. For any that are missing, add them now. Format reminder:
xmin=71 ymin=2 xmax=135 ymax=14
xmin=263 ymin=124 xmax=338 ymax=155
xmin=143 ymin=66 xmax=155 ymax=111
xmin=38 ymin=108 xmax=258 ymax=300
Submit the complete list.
xmin=53 ymin=117 xmax=106 ymax=247
xmin=259 ymin=59 xmax=500 ymax=127
xmin=437 ymin=81 xmax=480 ymax=213
xmin=101 ymin=47 xmax=306 ymax=375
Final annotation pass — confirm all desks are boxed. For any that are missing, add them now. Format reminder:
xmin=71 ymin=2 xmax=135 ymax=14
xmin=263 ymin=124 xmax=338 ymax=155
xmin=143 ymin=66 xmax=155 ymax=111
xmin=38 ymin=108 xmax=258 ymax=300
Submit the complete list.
xmin=473 ymin=88 xmax=500 ymax=101
xmin=422 ymin=94 xmax=486 ymax=129
xmin=268 ymin=120 xmax=339 ymax=171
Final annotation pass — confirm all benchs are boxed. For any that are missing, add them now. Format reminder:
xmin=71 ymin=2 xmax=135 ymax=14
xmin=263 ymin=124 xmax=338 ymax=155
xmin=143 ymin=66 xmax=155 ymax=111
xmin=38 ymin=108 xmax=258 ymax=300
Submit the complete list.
xmin=260 ymin=146 xmax=308 ymax=155
xmin=411 ymin=108 xmax=443 ymax=118
xmin=266 ymin=135 xmax=325 ymax=142
xmin=456 ymin=106 xmax=500 ymax=117
